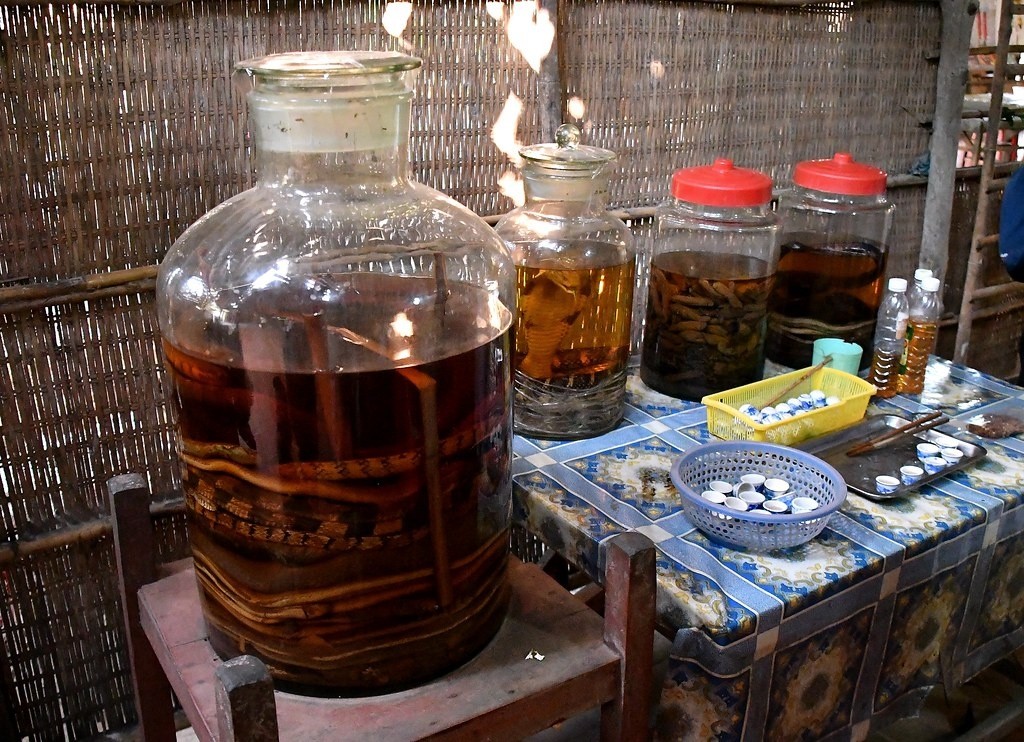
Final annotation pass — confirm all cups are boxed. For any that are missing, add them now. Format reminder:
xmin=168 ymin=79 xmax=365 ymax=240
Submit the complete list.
xmin=811 ymin=337 xmax=864 ymax=376
xmin=701 ymin=472 xmax=821 ymax=535
xmin=739 ymin=390 xmax=841 ymax=444
xmin=875 ymin=437 xmax=964 ymax=496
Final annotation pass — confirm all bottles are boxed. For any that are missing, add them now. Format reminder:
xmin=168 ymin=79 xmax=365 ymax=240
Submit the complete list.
xmin=491 ymin=123 xmax=636 ymax=440
xmin=868 ymin=278 xmax=909 ymax=398
xmin=639 ymin=159 xmax=784 ymax=405
xmin=764 ymin=153 xmax=897 ymax=373
xmin=906 ymin=268 xmax=934 ymax=307
xmin=896 ymin=278 xmax=941 ymax=393
xmin=153 ymin=47 xmax=515 ymax=701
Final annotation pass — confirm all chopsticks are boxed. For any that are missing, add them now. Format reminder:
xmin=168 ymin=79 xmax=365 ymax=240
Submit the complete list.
xmin=846 ymin=411 xmax=949 ymax=458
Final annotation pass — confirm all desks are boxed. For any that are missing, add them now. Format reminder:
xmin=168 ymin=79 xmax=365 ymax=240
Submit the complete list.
xmin=513 ymin=353 xmax=1024 ymax=742
